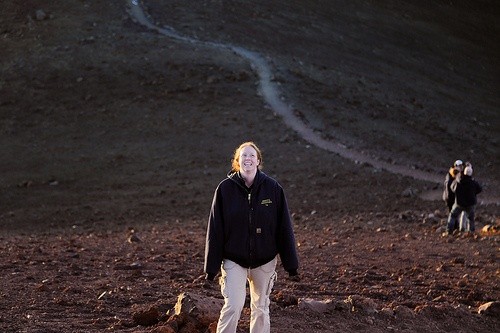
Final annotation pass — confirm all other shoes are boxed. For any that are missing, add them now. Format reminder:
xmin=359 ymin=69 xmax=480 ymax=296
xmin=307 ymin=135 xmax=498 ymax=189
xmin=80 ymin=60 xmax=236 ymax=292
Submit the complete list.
xmin=441 ymin=230 xmax=451 ymax=237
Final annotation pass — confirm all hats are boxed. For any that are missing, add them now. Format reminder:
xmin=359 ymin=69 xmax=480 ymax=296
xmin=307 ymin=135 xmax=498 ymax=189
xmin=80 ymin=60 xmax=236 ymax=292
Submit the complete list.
xmin=454 ymin=160 xmax=462 ymax=166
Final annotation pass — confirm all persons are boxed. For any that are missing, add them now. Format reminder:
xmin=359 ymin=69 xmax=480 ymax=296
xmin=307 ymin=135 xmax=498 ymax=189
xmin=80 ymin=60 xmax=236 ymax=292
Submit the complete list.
xmin=201 ymin=140 xmax=300 ymax=333
xmin=442 ymin=159 xmax=482 ymax=238
